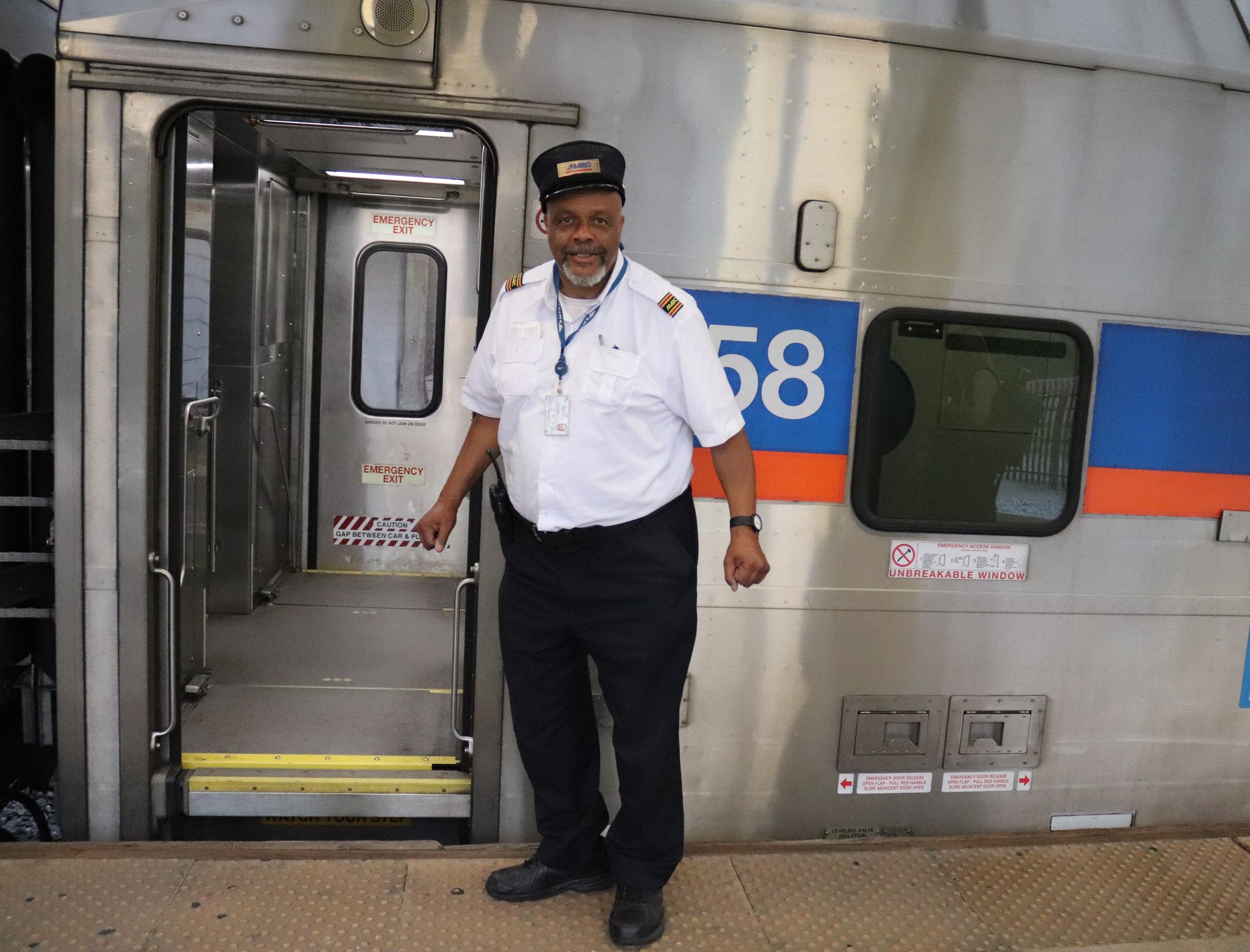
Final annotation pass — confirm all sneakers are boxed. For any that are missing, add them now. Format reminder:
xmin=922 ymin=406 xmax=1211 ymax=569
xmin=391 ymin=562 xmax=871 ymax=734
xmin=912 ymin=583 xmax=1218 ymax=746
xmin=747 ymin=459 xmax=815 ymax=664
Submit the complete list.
xmin=486 ymin=855 xmax=608 ymax=902
xmin=609 ymin=886 xmax=666 ymax=946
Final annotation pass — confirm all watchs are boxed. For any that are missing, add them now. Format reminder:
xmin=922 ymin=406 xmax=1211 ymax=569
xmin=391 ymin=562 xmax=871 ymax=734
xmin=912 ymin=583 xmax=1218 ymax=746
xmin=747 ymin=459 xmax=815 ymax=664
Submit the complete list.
xmin=730 ymin=513 xmax=763 ymax=534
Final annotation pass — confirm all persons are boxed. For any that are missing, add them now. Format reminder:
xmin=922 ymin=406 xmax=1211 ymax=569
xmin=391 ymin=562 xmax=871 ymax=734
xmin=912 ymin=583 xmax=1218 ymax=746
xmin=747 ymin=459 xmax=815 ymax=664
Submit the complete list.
xmin=415 ymin=140 xmax=771 ymax=948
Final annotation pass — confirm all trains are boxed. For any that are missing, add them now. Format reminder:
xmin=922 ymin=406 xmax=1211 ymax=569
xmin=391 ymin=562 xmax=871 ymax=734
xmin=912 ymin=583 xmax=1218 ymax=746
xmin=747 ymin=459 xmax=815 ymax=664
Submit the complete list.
xmin=0 ymin=1 xmax=1249 ymax=846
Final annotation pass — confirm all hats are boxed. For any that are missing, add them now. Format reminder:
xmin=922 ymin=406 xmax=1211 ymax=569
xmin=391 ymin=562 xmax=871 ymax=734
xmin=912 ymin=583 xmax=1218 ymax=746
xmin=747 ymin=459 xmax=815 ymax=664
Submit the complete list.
xmin=531 ymin=140 xmax=626 ymax=214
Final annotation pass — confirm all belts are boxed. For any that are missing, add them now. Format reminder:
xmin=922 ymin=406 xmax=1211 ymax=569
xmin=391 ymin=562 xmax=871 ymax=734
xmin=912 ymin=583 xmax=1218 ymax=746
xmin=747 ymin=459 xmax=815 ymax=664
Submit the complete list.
xmin=509 ymin=482 xmax=692 ymax=544
